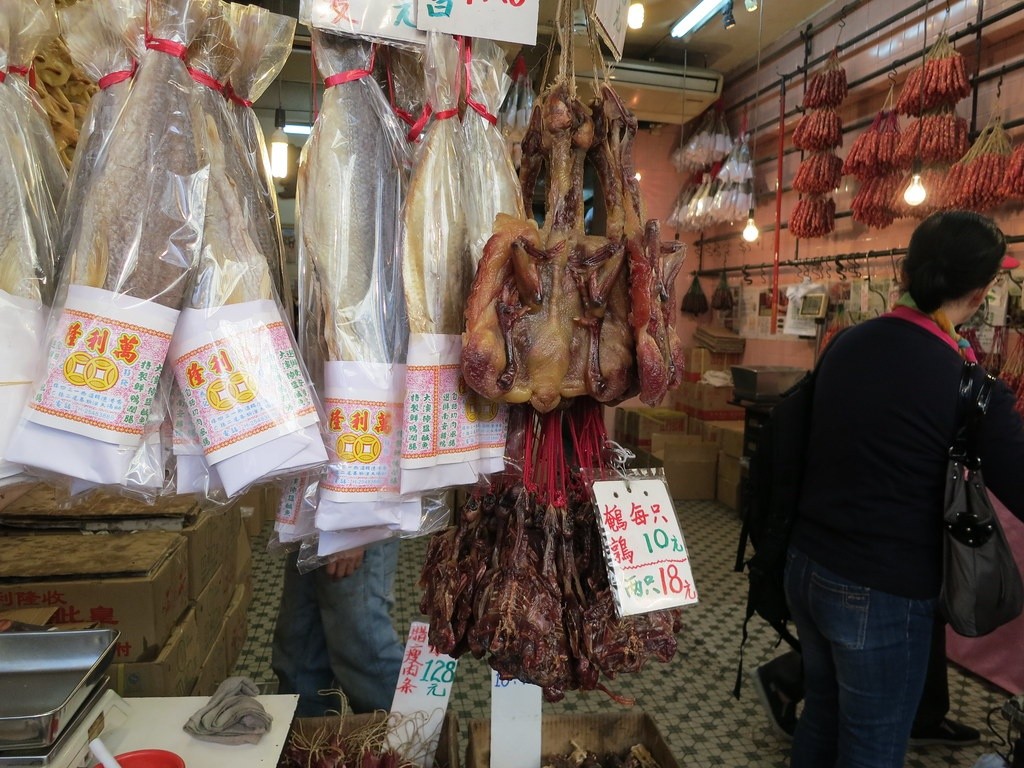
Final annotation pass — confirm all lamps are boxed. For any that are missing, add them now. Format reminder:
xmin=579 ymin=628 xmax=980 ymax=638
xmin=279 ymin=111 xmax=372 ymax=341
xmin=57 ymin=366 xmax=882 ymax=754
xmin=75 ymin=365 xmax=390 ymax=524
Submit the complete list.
xmin=670 ymin=0 xmax=759 ymax=39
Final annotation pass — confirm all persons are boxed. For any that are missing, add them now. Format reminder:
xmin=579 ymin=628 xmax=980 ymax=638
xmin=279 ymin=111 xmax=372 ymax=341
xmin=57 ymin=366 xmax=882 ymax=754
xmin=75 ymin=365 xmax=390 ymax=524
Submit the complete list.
xmin=758 ymin=209 xmax=1024 ymax=768
xmin=271 ymin=539 xmax=404 ymax=716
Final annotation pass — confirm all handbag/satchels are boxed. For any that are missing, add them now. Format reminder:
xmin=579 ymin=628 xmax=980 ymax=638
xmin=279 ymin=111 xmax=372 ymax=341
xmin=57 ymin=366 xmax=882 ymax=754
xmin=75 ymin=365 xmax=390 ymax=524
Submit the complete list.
xmin=744 ymin=327 xmax=846 ymax=570
xmin=939 ymin=363 xmax=1024 ymax=638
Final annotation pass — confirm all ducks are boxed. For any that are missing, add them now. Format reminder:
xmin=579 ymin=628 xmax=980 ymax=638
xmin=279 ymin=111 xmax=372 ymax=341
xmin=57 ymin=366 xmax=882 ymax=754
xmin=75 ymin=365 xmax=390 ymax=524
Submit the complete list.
xmin=585 ymin=84 xmax=685 ymax=406
xmin=462 ymin=84 xmax=633 ymax=415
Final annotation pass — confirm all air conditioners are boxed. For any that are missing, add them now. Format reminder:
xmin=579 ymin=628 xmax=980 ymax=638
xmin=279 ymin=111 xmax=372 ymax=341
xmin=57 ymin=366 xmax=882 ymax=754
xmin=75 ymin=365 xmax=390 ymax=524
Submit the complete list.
xmin=542 ymin=56 xmax=723 ymax=125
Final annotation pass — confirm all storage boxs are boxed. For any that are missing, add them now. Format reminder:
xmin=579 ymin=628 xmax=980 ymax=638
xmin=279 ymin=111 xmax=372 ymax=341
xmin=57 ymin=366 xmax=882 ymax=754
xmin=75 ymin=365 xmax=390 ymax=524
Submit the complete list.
xmin=273 ymin=708 xmax=461 ymax=768
xmin=469 ymin=710 xmax=683 ymax=768
xmin=611 ymin=346 xmax=749 ymax=508
xmin=0 ymin=480 xmax=275 ymax=701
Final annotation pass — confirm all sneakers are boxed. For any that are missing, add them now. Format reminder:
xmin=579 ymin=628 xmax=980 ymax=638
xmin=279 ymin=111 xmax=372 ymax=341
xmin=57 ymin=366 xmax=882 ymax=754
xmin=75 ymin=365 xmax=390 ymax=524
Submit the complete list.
xmin=909 ymin=711 xmax=979 ymax=749
xmin=752 ymin=663 xmax=802 ymax=740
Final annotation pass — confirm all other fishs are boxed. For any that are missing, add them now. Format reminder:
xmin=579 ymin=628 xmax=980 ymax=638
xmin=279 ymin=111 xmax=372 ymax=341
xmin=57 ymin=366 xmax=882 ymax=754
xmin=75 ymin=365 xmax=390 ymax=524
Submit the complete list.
xmin=1 ymin=0 xmax=527 ymax=366
